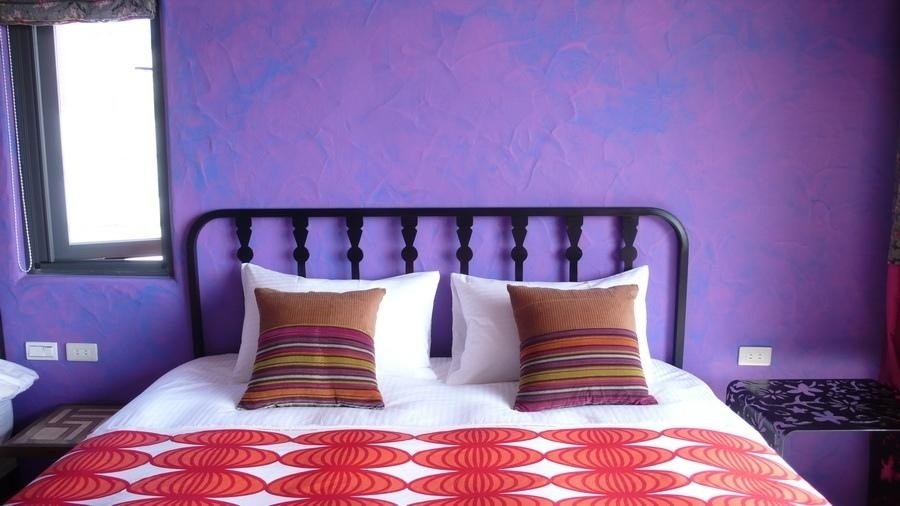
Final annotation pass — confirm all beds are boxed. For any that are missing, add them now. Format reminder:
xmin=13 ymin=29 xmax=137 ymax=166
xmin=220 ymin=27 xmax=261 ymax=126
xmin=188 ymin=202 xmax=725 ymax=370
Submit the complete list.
xmin=4 ymin=206 xmax=831 ymax=506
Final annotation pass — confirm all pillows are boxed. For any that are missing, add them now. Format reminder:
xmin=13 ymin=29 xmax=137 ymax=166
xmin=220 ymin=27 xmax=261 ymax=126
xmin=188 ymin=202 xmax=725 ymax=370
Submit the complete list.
xmin=504 ymin=282 xmax=655 ymax=413
xmin=234 ymin=256 xmax=440 ymax=380
xmin=239 ymin=286 xmax=387 ymax=411
xmin=448 ymin=267 xmax=658 ymax=392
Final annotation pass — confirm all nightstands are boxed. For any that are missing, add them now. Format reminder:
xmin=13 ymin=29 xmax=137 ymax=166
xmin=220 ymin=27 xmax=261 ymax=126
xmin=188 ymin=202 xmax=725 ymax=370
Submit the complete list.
xmin=727 ymin=379 xmax=900 ymax=506
xmin=1 ymin=398 xmax=123 ymax=499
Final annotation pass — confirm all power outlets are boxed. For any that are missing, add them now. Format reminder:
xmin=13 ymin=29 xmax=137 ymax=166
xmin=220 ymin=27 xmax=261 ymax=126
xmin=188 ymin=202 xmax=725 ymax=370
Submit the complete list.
xmin=738 ymin=346 xmax=772 ymax=367
xmin=24 ymin=343 xmax=60 ymax=361
xmin=65 ymin=342 xmax=99 ymax=362
